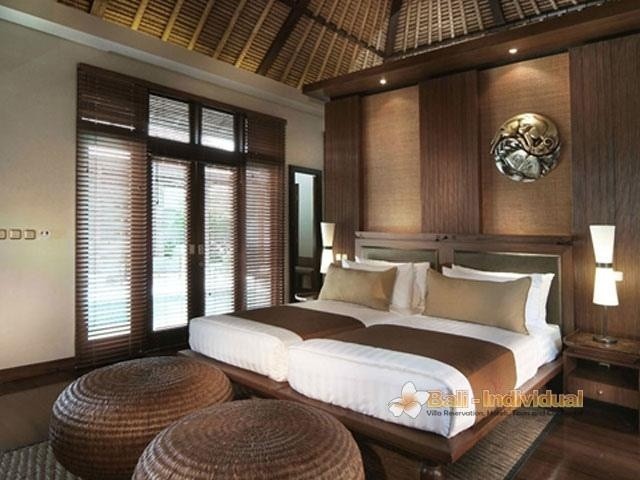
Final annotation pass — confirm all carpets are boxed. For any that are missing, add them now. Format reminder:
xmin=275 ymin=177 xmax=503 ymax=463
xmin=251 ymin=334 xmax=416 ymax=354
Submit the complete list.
xmin=0 ymin=381 xmax=570 ymax=480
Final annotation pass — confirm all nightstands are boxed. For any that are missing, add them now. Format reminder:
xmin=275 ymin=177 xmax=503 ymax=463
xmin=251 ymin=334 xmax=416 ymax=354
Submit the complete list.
xmin=563 ymin=330 xmax=640 ymax=417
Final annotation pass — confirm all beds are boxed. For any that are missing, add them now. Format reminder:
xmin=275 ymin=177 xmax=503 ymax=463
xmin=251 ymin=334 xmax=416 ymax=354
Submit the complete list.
xmin=175 ymin=230 xmax=581 ymax=480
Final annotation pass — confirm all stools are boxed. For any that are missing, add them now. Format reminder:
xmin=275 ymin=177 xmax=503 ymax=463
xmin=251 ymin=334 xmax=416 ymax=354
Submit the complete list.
xmin=49 ymin=353 xmax=365 ymax=479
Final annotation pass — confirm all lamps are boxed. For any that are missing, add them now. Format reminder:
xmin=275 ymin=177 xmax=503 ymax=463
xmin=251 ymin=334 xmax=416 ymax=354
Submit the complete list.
xmin=319 ymin=221 xmax=335 ymax=275
xmin=589 ymin=224 xmax=619 ymax=345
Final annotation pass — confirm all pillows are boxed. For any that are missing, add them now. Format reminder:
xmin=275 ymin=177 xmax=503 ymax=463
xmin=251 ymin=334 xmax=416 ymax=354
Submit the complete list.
xmin=314 ymin=255 xmax=555 ymax=336
